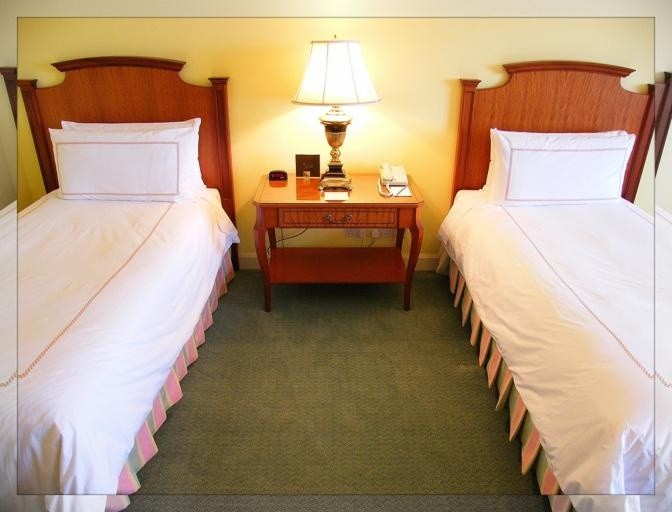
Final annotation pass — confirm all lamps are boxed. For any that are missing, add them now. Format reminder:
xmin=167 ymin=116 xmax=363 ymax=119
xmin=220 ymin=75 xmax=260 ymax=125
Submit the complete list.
xmin=291 ymin=36 xmax=381 ymax=191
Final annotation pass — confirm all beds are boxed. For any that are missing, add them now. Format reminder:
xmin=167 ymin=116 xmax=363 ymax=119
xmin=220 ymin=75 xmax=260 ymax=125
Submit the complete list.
xmin=451 ymin=60 xmax=671 ymax=511
xmin=15 ymin=55 xmax=238 ymax=449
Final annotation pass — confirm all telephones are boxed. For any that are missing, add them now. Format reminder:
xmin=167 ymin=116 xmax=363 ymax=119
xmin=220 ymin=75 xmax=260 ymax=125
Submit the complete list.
xmin=378 ymin=164 xmax=409 ymax=185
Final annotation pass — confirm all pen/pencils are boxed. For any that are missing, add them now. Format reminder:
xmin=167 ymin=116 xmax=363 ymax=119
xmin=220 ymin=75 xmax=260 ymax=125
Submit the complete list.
xmin=391 ymin=186 xmax=408 ymax=199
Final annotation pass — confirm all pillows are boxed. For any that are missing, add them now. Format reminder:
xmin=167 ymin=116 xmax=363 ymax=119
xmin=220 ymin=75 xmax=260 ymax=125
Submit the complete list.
xmin=48 ymin=116 xmax=204 ymax=203
xmin=484 ymin=128 xmax=636 ymax=206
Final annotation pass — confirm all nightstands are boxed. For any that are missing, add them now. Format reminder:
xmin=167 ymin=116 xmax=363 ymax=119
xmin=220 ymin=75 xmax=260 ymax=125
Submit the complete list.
xmin=253 ymin=172 xmax=423 ymax=311
